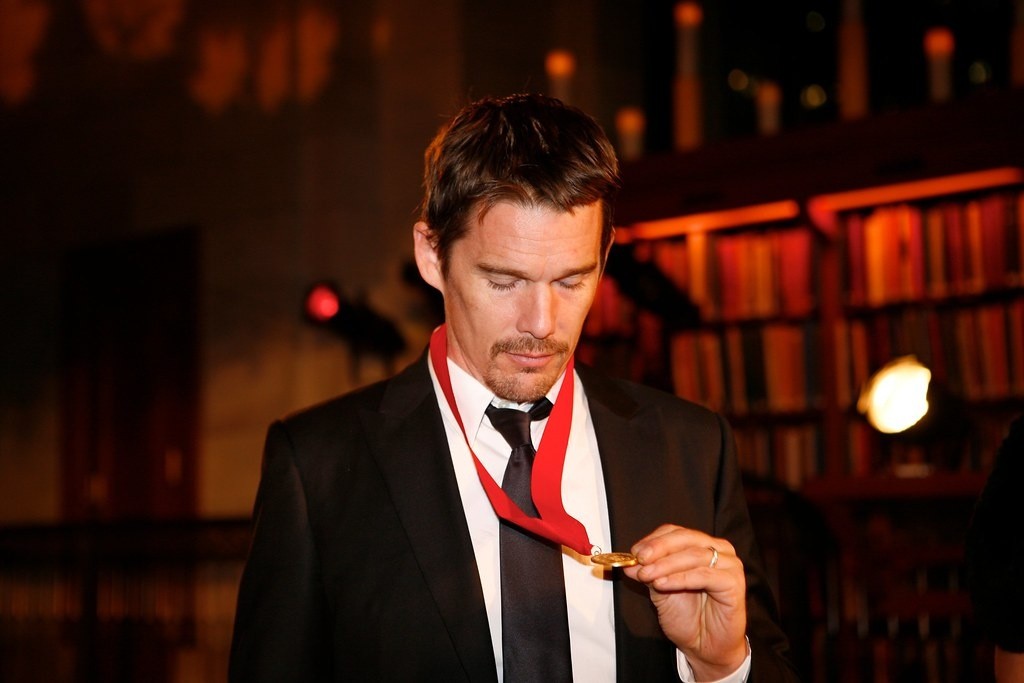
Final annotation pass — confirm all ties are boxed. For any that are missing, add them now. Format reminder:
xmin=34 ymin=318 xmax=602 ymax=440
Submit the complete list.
xmin=485 ymin=397 xmax=573 ymax=683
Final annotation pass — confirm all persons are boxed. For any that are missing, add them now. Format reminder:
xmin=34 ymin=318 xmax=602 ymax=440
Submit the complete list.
xmin=223 ymin=90 xmax=787 ymax=682
xmin=968 ymin=413 xmax=1024 ymax=682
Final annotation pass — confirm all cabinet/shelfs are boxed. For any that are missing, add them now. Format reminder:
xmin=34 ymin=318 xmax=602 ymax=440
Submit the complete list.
xmin=571 ymin=166 xmax=1024 ymax=683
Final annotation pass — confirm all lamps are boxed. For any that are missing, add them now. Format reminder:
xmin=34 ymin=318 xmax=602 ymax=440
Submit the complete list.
xmin=300 ymin=278 xmax=404 ymax=364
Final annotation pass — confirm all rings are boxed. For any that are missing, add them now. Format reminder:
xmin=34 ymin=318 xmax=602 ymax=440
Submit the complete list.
xmin=707 ymin=546 xmax=718 ymax=567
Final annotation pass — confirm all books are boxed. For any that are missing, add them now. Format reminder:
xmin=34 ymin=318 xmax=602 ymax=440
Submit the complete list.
xmin=573 ymin=185 xmax=1024 ymax=683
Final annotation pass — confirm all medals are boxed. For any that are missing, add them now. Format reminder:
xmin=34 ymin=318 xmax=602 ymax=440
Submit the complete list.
xmin=590 ymin=551 xmax=637 ymax=567
